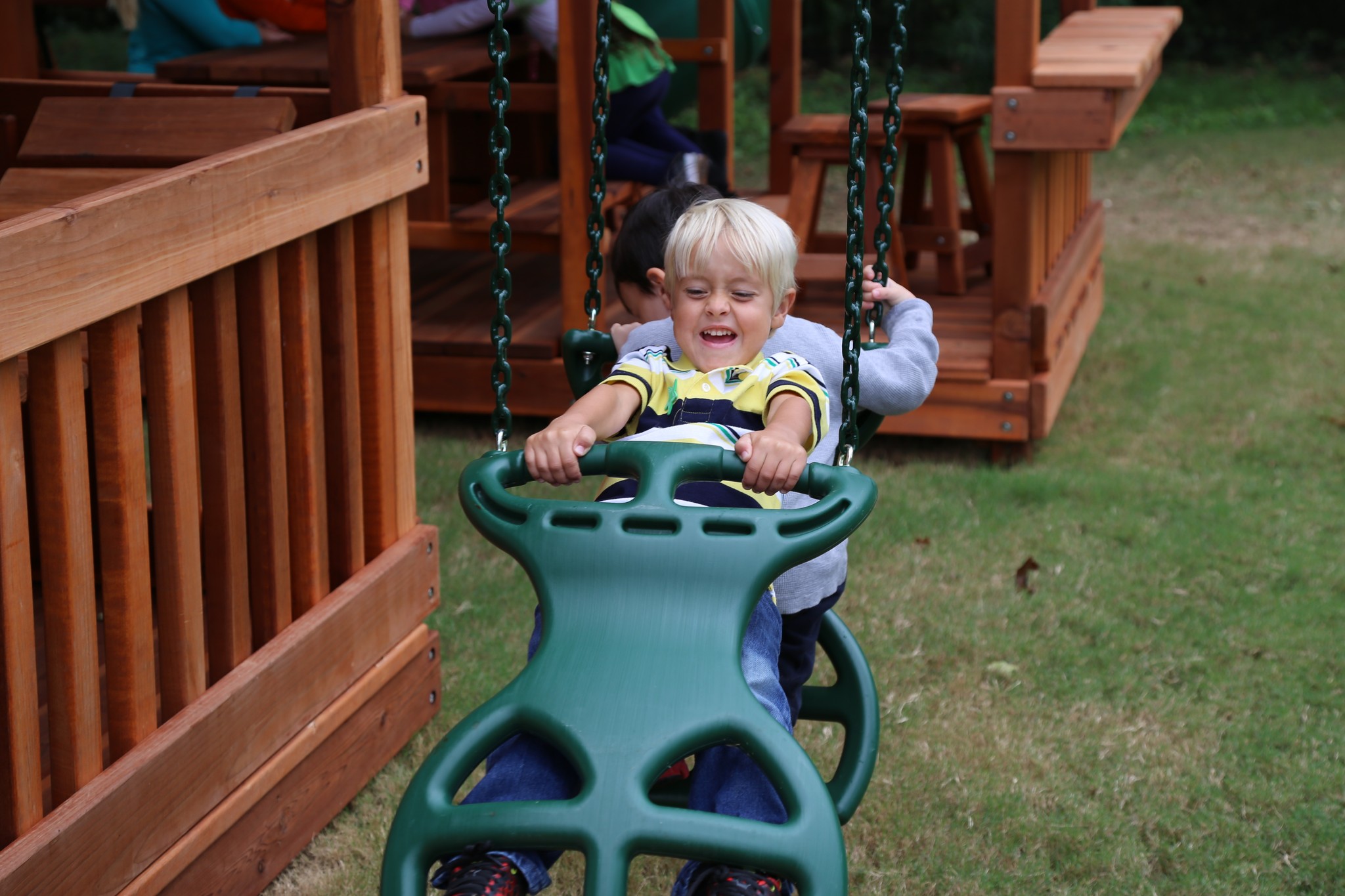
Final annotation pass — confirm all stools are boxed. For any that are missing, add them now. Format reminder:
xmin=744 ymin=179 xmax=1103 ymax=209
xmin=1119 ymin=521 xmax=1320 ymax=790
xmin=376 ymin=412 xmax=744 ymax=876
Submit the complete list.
xmin=870 ymin=89 xmax=993 ymax=293
xmin=776 ymin=110 xmax=909 ymax=297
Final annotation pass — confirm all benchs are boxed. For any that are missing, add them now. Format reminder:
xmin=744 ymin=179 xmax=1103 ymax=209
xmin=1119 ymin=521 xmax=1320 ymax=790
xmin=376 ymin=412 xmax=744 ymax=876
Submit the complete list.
xmin=454 ymin=169 xmax=656 ymax=254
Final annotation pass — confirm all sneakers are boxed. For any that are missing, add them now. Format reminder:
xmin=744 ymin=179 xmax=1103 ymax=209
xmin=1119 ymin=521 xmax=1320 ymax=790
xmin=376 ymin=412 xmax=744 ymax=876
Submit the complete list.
xmin=445 ymin=855 xmax=527 ymax=896
xmin=686 ymin=866 xmax=783 ymax=896
xmin=650 ymin=757 xmax=689 ymax=801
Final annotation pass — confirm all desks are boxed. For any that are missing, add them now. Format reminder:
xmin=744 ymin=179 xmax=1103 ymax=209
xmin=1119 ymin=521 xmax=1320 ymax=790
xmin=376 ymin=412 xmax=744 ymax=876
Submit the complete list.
xmin=155 ymin=32 xmax=535 ymax=245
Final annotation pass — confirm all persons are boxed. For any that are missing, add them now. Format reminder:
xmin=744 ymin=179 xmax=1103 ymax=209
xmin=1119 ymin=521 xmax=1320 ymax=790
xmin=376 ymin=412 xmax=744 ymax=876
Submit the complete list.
xmin=608 ymin=182 xmax=940 ymax=797
xmin=432 ymin=200 xmax=831 ymax=896
xmin=33 ymin=0 xmax=725 ymax=216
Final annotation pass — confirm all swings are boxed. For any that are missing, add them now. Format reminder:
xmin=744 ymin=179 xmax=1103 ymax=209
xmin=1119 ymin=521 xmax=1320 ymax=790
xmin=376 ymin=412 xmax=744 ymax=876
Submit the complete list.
xmin=372 ymin=15 xmax=915 ymax=896
